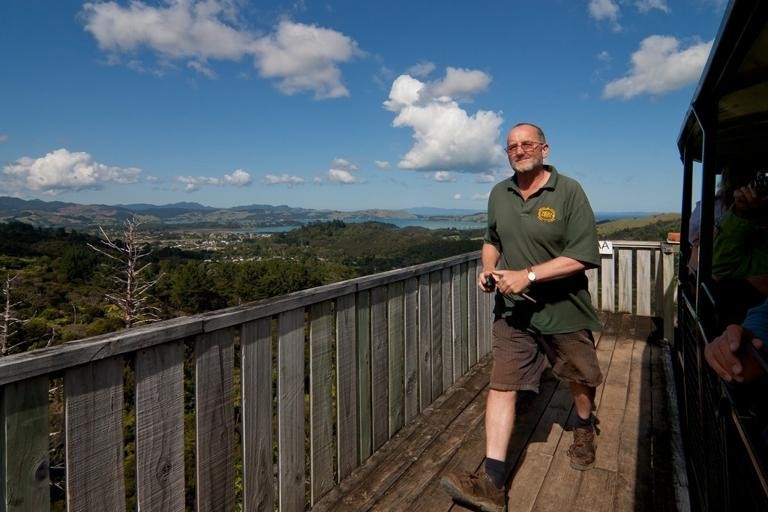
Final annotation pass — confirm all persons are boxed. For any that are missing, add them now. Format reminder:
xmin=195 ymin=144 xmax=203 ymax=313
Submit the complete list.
xmin=439 ymin=123 xmax=607 ymax=512
xmin=687 ymin=154 xmax=766 ymax=385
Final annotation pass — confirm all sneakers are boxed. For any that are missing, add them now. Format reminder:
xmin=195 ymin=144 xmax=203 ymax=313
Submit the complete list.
xmin=569 ymin=428 xmax=596 ymax=471
xmin=442 ymin=472 xmax=507 ymax=512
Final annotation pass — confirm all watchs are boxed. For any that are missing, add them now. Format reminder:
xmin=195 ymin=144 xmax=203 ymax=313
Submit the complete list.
xmin=527 ymin=265 xmax=538 ymax=284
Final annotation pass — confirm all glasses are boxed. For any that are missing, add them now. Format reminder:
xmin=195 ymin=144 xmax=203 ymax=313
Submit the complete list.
xmin=504 ymin=142 xmax=543 ymax=154
